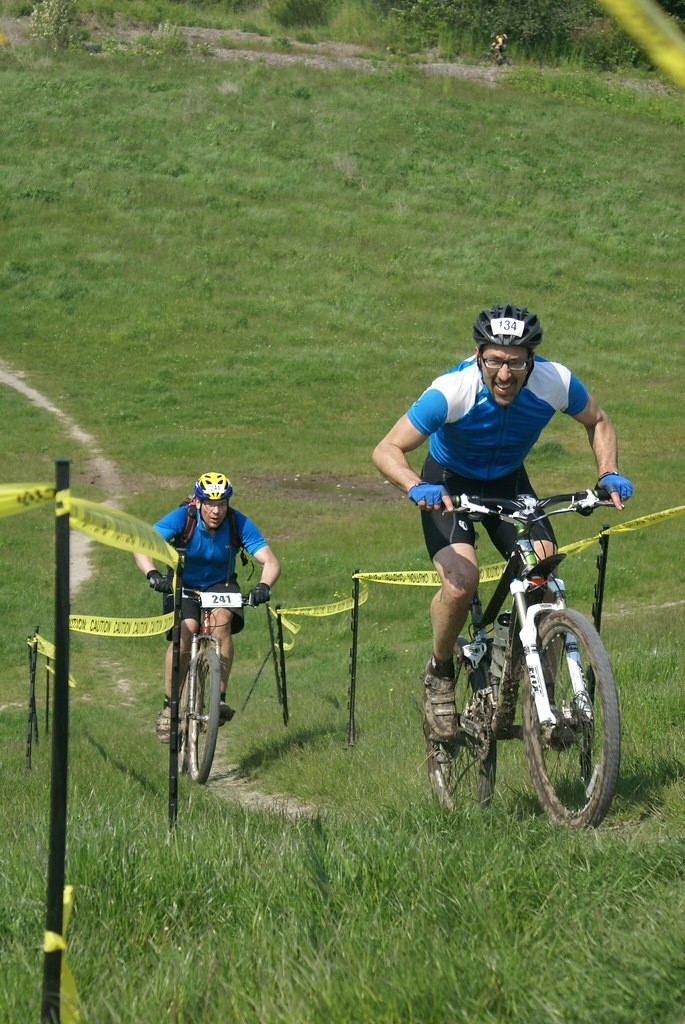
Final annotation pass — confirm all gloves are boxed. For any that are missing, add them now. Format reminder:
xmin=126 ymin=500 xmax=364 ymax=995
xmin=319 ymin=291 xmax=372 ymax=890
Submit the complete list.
xmin=147 ymin=570 xmax=170 ymax=592
xmin=247 ymin=582 xmax=270 ymax=607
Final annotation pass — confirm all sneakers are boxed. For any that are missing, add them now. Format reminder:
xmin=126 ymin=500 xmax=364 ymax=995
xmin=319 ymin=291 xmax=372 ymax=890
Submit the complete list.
xmin=419 ymin=657 xmax=458 ymax=738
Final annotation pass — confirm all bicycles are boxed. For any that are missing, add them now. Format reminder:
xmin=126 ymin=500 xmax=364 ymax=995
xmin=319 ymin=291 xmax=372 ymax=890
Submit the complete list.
xmin=146 ymin=580 xmax=255 ymax=785
xmin=424 ymin=488 xmax=627 ymax=830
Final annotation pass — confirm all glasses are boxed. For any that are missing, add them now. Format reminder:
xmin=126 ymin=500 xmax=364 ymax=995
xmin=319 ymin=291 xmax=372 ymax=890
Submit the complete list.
xmin=203 ymin=502 xmax=226 ymax=509
xmin=481 ymin=356 xmax=529 ymax=371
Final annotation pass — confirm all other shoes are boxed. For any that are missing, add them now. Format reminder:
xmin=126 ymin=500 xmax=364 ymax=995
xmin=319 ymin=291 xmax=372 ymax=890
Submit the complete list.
xmin=219 ymin=701 xmax=235 ymax=727
xmin=156 ymin=706 xmax=172 ymax=744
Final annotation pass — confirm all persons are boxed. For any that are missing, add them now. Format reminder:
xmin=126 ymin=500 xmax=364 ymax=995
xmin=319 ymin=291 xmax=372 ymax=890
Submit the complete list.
xmin=372 ymin=305 xmax=632 ymax=742
xmin=133 ymin=473 xmax=280 ymax=743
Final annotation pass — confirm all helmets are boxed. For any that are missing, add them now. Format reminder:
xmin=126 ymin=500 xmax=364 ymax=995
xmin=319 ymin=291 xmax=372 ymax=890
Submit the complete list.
xmin=472 ymin=304 xmax=543 ymax=347
xmin=194 ymin=472 xmax=233 ymax=500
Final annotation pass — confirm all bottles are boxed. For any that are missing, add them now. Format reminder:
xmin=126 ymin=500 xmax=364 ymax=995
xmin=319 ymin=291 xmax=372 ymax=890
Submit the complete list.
xmin=490 ymin=608 xmax=511 ymax=678
